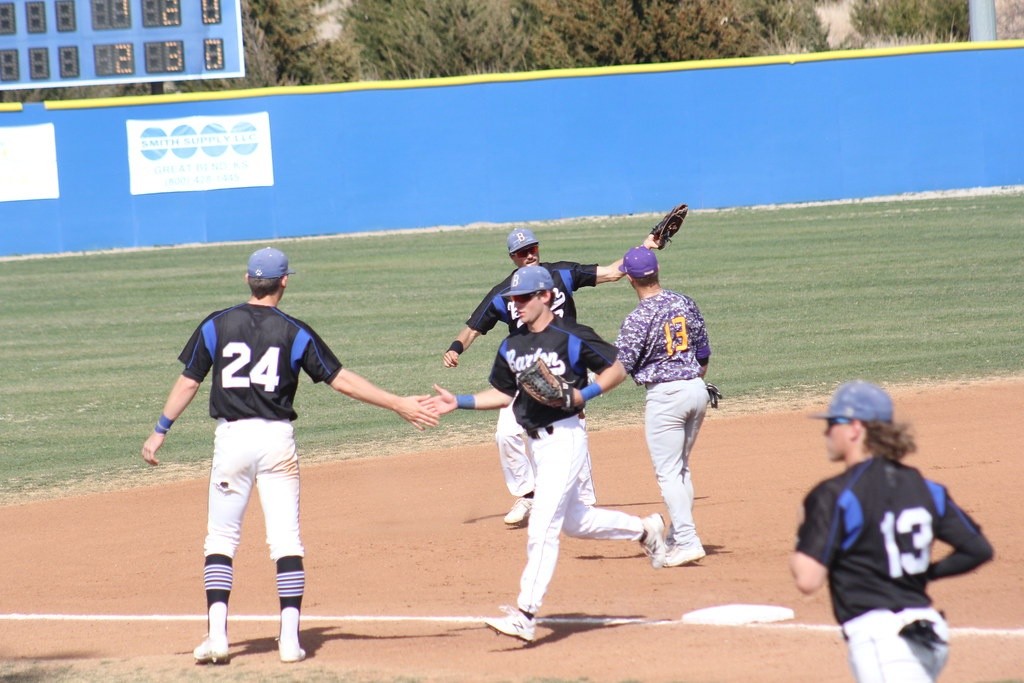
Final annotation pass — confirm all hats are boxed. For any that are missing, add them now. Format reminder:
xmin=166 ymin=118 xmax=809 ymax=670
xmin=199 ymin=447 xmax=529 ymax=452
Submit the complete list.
xmin=247 ymin=246 xmax=295 ymax=280
xmin=810 ymin=381 xmax=894 ymax=425
xmin=502 ymin=265 xmax=554 ymax=296
xmin=506 ymin=228 xmax=539 ymax=254
xmin=618 ymin=246 xmax=658 ymax=278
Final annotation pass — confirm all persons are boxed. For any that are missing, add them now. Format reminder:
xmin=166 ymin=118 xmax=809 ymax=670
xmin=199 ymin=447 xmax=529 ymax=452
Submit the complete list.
xmin=420 ymin=264 xmax=666 ymax=644
xmin=444 ymin=203 xmax=689 ymax=524
xmin=517 ymin=245 xmax=711 ymax=568
xmin=142 ymin=247 xmax=442 ymax=663
xmin=790 ymin=380 xmax=993 ymax=683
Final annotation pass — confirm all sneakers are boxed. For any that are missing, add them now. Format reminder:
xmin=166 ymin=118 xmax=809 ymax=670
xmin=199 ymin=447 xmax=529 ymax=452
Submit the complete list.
xmin=278 ymin=641 xmax=306 ymax=662
xmin=664 ymin=539 xmax=705 ymax=566
xmin=637 ymin=514 xmax=666 ymax=569
xmin=193 ymin=635 xmax=229 ymax=662
xmin=504 ymin=497 xmax=535 ymax=525
xmin=485 ymin=604 xmax=536 ymax=642
xmin=664 ymin=527 xmax=676 ymax=545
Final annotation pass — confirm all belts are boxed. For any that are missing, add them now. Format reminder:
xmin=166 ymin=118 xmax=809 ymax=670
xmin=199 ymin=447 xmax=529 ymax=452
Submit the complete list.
xmin=226 ymin=416 xmax=237 ymax=422
xmin=528 ymin=411 xmax=585 ymax=440
xmin=891 ymin=604 xmax=945 ymax=618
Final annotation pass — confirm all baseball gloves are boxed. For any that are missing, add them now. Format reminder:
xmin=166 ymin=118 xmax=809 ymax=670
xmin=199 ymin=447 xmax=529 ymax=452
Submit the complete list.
xmin=649 ymin=203 xmax=689 ymax=251
xmin=517 ymin=356 xmax=575 ymax=415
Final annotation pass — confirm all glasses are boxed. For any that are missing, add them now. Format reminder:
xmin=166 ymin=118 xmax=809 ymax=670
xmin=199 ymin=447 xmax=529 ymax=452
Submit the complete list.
xmin=512 ymin=245 xmax=539 ymax=259
xmin=512 ymin=290 xmax=545 ymax=303
xmin=825 ymin=415 xmax=854 ymax=427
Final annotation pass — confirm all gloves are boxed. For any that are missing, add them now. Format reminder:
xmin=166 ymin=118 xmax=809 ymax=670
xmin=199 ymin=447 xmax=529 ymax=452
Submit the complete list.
xmin=705 ymin=382 xmax=722 ymax=409
xmin=900 ymin=618 xmax=948 ymax=651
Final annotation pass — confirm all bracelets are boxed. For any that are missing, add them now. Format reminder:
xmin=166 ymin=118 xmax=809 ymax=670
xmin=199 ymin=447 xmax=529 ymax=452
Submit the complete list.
xmin=445 ymin=340 xmax=464 ymax=355
xmin=580 ymin=382 xmax=602 ymax=402
xmin=456 ymin=394 xmax=475 ymax=409
xmin=154 ymin=411 xmax=174 ymax=434
